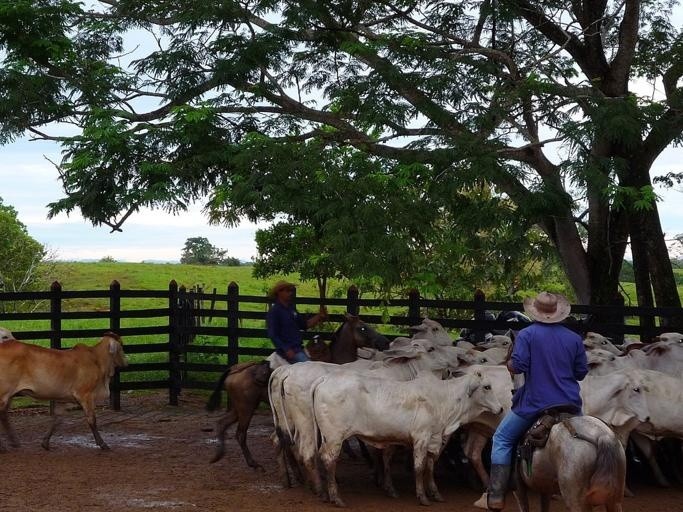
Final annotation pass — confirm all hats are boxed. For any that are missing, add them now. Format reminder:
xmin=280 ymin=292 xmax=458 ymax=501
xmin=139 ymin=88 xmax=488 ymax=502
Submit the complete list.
xmin=523 ymin=291 xmax=571 ymax=324
xmin=269 ymin=280 xmax=298 ymax=297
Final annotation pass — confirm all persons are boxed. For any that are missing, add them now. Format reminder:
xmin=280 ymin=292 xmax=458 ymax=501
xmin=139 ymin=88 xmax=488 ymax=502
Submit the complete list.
xmin=474 ymin=292 xmax=588 ymax=510
xmin=266 ymin=277 xmax=329 ymax=364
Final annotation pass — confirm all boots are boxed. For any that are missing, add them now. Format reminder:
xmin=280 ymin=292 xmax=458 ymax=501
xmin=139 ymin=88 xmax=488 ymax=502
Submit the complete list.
xmin=488 ymin=463 xmax=513 ymax=509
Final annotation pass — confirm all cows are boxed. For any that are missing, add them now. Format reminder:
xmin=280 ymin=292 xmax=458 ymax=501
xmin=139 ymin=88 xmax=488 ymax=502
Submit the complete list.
xmin=0 ymin=331 xmax=128 ymax=454
xmin=267 ymin=309 xmax=682 ymax=511
xmin=0 ymin=325 xmax=14 ymax=344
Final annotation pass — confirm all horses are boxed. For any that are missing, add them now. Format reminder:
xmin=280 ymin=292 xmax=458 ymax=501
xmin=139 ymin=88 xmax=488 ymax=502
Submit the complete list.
xmin=205 ymin=310 xmax=391 ymax=474
